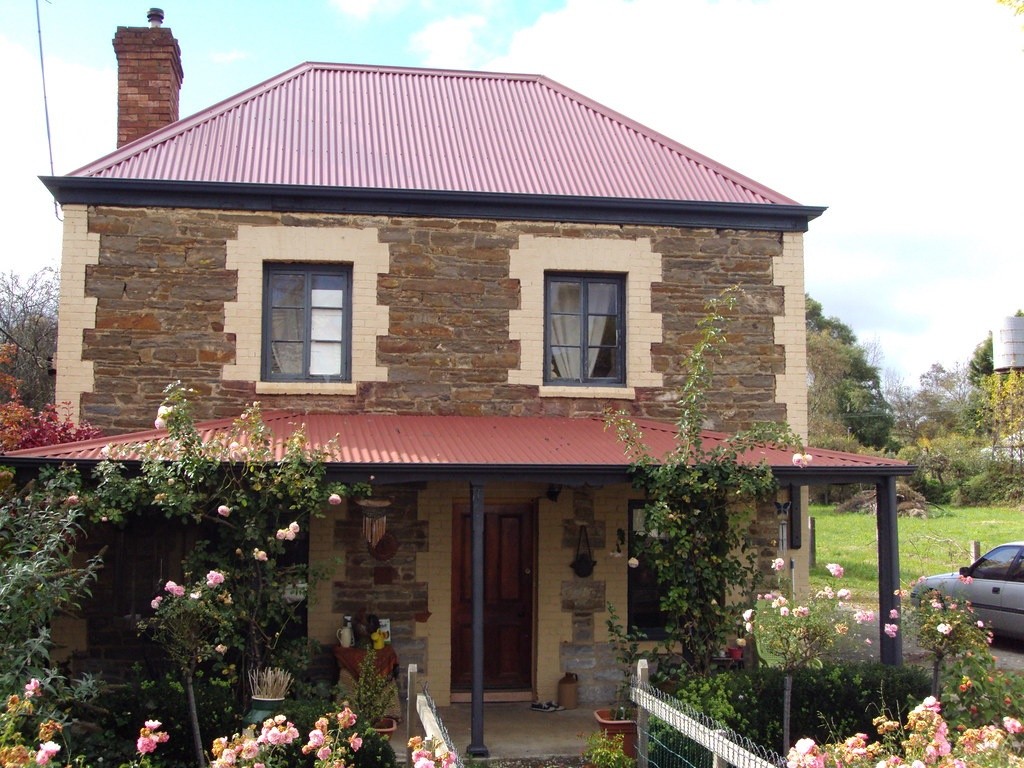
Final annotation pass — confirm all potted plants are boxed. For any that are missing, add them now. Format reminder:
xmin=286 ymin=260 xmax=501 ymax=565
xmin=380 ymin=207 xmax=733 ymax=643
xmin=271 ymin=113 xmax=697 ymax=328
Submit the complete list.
xmin=594 ymin=600 xmax=641 ymax=758
xmin=354 ymin=648 xmax=399 ymax=741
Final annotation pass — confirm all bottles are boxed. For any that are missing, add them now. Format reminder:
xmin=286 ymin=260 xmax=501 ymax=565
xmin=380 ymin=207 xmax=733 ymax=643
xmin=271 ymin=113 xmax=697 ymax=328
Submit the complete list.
xmin=558 ymin=672 xmax=578 ymax=709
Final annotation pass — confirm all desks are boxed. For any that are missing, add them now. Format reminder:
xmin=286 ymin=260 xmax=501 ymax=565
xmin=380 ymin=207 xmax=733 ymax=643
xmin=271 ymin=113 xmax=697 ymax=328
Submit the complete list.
xmin=334 ymin=645 xmax=397 ymax=682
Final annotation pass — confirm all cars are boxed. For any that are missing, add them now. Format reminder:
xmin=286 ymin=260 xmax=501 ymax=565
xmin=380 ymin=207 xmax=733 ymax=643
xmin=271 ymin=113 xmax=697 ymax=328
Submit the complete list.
xmin=909 ymin=540 xmax=1024 ymax=644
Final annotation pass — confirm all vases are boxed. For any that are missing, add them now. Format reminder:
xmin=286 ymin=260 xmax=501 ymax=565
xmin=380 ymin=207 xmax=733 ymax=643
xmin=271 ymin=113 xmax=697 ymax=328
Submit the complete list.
xmin=730 ymin=648 xmax=743 ymax=658
xmin=242 ymin=695 xmax=286 ymax=731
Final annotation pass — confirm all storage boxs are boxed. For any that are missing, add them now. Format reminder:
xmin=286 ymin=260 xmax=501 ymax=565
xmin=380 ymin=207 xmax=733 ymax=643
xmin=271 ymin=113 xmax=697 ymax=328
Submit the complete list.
xmin=379 ymin=619 xmax=391 ymax=643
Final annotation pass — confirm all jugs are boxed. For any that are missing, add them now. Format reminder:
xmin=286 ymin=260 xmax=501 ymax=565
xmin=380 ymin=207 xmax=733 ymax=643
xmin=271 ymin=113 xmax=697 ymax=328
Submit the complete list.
xmin=371 ymin=628 xmax=385 ymax=649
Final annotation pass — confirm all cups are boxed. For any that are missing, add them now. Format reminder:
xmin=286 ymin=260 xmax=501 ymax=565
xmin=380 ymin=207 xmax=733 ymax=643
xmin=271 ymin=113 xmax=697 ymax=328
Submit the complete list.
xmin=335 ymin=627 xmax=353 ymax=648
